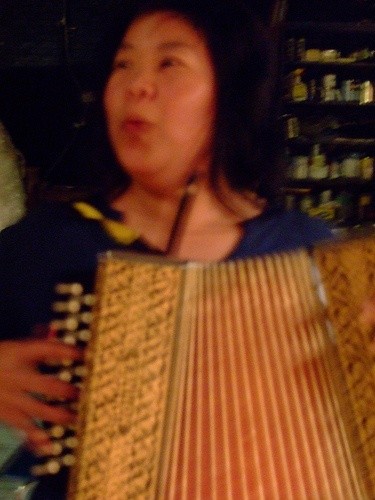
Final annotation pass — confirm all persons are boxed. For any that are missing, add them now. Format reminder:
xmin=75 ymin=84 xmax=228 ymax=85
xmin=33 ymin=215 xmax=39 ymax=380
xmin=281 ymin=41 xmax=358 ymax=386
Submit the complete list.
xmin=1 ymin=1 xmax=374 ymax=499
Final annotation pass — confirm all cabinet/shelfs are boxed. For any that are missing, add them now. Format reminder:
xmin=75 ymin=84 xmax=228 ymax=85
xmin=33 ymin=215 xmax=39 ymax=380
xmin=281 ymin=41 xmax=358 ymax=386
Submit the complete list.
xmin=269 ymin=38 xmax=375 ymax=223
